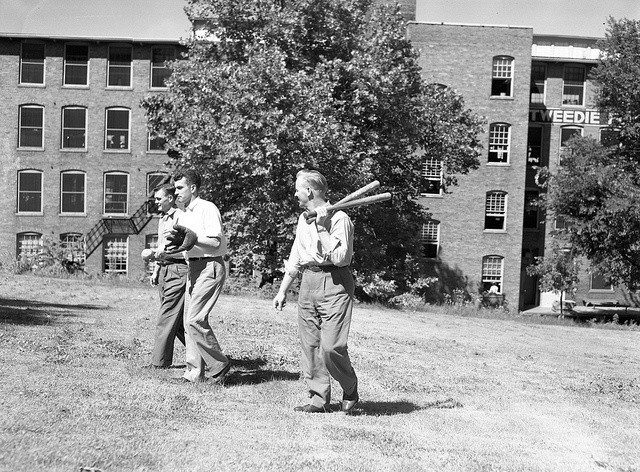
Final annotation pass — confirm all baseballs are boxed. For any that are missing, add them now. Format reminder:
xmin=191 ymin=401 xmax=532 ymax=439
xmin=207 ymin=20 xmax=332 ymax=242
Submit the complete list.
xmin=142 ymin=249 xmax=154 ymax=259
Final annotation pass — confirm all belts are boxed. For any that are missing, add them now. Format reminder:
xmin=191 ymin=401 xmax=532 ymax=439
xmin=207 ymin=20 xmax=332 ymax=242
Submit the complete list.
xmin=304 ymin=265 xmax=348 ymax=272
xmin=158 ymin=259 xmax=185 ymax=266
xmin=187 ymin=257 xmax=212 ymax=260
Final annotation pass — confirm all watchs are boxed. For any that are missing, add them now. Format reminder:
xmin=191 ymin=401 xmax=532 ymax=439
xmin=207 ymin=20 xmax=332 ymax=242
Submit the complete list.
xmin=316 ymin=226 xmax=327 ymax=233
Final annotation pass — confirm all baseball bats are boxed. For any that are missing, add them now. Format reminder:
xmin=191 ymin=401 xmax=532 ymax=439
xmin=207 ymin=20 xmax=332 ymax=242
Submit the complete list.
xmin=306 ymin=179 xmax=380 ymax=225
xmin=303 ymin=192 xmax=392 ymax=219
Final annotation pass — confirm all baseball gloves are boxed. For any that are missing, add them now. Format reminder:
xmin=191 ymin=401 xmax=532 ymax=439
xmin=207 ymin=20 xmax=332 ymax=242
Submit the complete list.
xmin=163 ymin=225 xmax=198 ymax=254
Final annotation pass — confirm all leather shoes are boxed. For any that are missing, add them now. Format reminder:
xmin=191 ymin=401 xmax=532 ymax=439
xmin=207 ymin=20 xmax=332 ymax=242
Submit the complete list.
xmin=294 ymin=404 xmax=330 ymax=412
xmin=140 ymin=364 xmax=169 ymax=370
xmin=169 ymin=377 xmax=194 ymax=384
xmin=204 ymin=360 xmax=232 ymax=387
xmin=342 ymin=380 xmax=359 ymax=413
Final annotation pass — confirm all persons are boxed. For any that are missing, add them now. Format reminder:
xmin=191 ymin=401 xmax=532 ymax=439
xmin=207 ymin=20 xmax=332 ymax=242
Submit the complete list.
xmin=156 ymin=170 xmax=232 ymax=385
xmin=488 ymin=282 xmax=498 ymax=294
xmin=273 ymin=169 xmax=358 ymax=414
xmin=141 ymin=184 xmax=185 ymax=370
